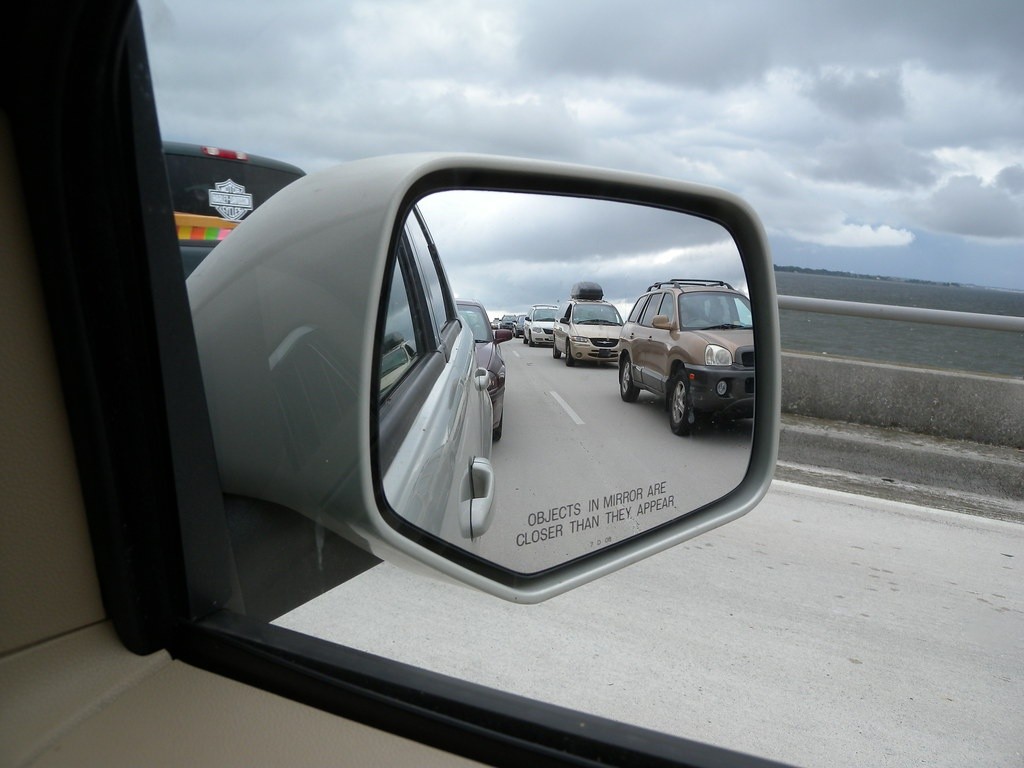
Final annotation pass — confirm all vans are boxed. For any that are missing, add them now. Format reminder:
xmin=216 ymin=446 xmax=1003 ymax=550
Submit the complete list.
xmin=161 ymin=140 xmax=305 ymax=282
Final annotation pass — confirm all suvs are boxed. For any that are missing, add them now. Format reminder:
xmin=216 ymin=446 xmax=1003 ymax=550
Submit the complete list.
xmin=616 ymin=279 xmax=754 ymax=436
xmin=553 ymin=297 xmax=624 ymax=367
xmin=491 ymin=304 xmax=559 ymax=347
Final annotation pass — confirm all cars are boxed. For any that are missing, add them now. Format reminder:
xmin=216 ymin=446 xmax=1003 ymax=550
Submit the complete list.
xmin=377 ymin=206 xmax=496 ymax=554
xmin=455 ymin=299 xmax=513 ymax=442
